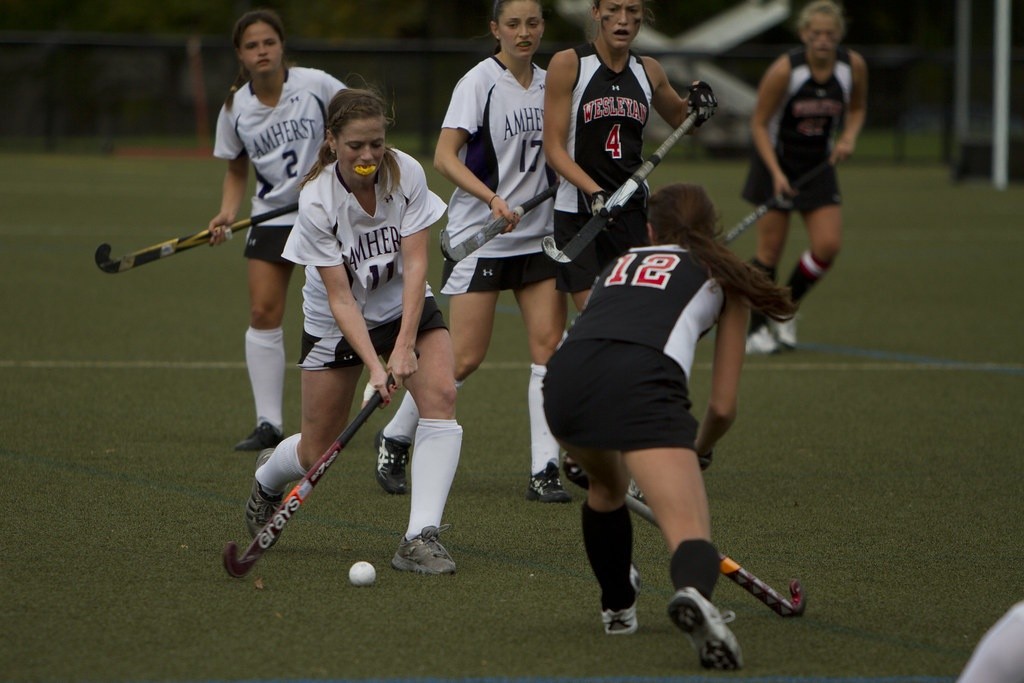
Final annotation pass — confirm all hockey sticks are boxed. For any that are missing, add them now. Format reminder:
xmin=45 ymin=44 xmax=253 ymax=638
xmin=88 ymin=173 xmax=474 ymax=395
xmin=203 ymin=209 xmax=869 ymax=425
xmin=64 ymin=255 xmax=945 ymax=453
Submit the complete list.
xmin=222 ymin=349 xmax=422 ymax=578
xmin=95 ymin=202 xmax=299 ymax=274
xmin=439 ymin=181 xmax=561 ymax=263
xmin=541 ymin=111 xmax=699 ymax=263
xmin=722 ymin=164 xmax=831 ymax=244
xmin=623 ymin=492 xmax=807 ymax=616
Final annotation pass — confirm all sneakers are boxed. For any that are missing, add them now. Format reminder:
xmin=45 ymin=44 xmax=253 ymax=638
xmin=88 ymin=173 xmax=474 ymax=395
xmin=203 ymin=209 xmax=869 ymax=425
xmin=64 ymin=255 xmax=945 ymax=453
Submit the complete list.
xmin=392 ymin=524 xmax=457 ymax=576
xmin=746 ymin=326 xmax=780 ymax=354
xmin=245 ymin=447 xmax=283 ymax=549
xmin=601 ymin=562 xmax=643 ymax=635
xmin=628 ymin=477 xmax=644 ymax=498
xmin=526 ymin=461 xmax=571 ymax=504
xmin=235 ymin=422 xmax=283 ymax=450
xmin=668 ymin=586 xmax=743 ymax=671
xmin=374 ymin=428 xmax=412 ymax=495
xmin=767 ymin=311 xmax=797 ymax=349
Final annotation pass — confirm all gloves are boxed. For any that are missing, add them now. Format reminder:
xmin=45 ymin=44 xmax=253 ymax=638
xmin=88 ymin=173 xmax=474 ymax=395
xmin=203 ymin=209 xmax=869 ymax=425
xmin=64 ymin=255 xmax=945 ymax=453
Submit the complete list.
xmin=591 ymin=190 xmax=622 ymax=232
xmin=686 ymin=81 xmax=718 ymax=127
xmin=698 ymin=449 xmax=713 ymax=471
xmin=562 ymin=452 xmax=580 ymax=478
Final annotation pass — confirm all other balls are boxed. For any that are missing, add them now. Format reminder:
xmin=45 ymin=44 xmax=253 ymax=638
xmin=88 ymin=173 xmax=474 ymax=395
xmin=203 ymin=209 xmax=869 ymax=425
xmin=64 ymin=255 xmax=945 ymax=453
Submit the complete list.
xmin=349 ymin=562 xmax=376 ymax=586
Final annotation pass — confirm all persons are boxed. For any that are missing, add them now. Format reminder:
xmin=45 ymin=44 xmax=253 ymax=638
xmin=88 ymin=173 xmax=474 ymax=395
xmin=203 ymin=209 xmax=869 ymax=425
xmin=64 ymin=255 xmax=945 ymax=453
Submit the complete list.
xmin=375 ymin=0 xmax=575 ymax=504
xmin=244 ymin=89 xmax=463 ymax=577
xmin=742 ymin=2 xmax=870 ymax=355
xmin=541 ymin=185 xmax=800 ymax=673
xmin=544 ymin=0 xmax=717 ymax=311
xmin=207 ymin=13 xmax=352 ymax=450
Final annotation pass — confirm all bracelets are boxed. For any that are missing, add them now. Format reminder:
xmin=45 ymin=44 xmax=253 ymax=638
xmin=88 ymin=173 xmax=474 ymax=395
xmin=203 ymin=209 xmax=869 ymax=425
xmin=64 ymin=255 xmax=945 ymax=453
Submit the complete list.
xmin=489 ymin=194 xmax=499 ymax=210
